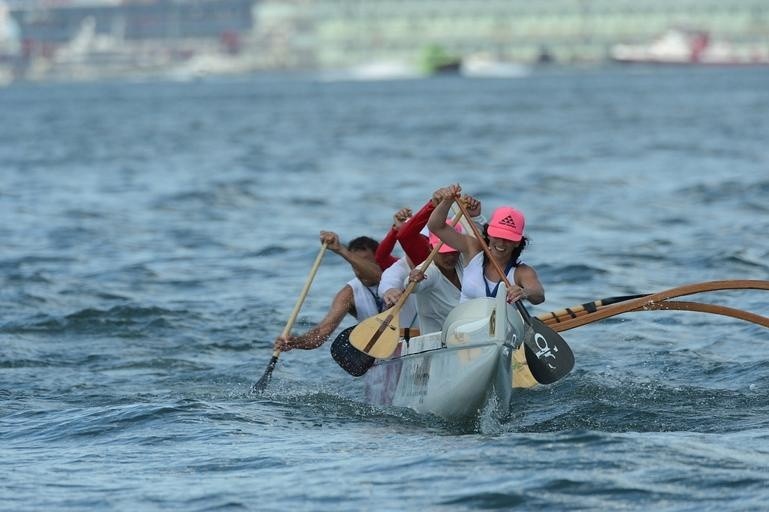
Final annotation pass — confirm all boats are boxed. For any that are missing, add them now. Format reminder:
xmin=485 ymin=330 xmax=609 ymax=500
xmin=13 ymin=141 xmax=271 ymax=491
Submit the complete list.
xmin=364 ymin=282 xmax=525 ymax=432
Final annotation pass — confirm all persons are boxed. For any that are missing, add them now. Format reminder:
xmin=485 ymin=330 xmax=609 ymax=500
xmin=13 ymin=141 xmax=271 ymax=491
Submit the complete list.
xmin=374 ymin=183 xmax=547 ymax=353
xmin=272 ymin=231 xmax=381 ymax=350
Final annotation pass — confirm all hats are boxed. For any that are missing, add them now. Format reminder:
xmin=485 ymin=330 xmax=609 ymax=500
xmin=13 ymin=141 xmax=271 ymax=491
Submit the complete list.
xmin=486 ymin=206 xmax=525 ymax=243
xmin=428 ymin=218 xmax=463 ymax=254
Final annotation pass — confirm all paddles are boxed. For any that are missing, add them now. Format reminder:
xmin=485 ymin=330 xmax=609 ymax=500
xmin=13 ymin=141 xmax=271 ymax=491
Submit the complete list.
xmin=249 ymin=240 xmax=328 ymax=397
xmin=450 ymin=198 xmax=540 ymax=387
xmin=449 ymin=186 xmax=575 ymax=385
xmin=349 ymin=197 xmax=470 ymax=360
xmin=331 ymin=269 xmax=409 ymax=377
